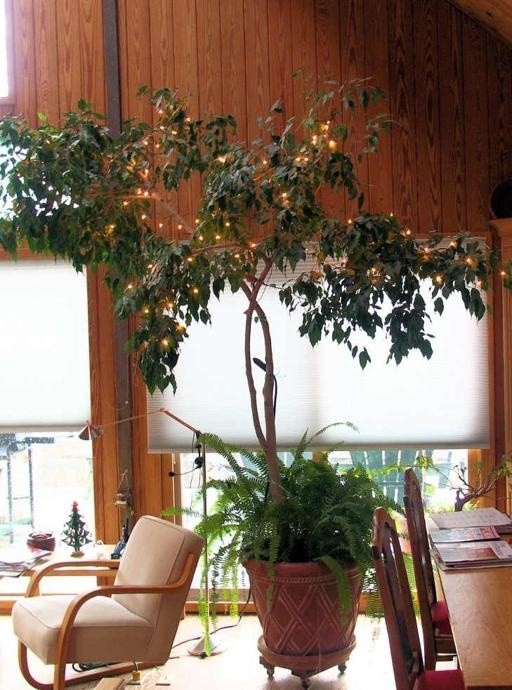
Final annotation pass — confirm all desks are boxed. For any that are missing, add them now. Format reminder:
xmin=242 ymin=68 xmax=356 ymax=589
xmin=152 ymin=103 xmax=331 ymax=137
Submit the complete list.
xmin=0 ymin=544 xmax=122 ymax=617
xmin=426 ymin=513 xmax=512 ymax=689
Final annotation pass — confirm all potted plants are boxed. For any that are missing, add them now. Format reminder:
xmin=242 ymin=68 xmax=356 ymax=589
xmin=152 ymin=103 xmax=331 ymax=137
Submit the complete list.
xmin=157 ymin=420 xmax=424 ymax=690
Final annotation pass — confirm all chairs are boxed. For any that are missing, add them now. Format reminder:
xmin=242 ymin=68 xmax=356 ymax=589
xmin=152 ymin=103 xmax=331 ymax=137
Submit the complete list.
xmin=371 ymin=463 xmax=466 ymax=689
xmin=9 ymin=515 xmax=204 ymax=690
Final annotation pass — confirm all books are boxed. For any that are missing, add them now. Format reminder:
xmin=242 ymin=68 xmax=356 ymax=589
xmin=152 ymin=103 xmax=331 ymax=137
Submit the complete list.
xmin=428 ymin=507 xmax=512 ymax=570
